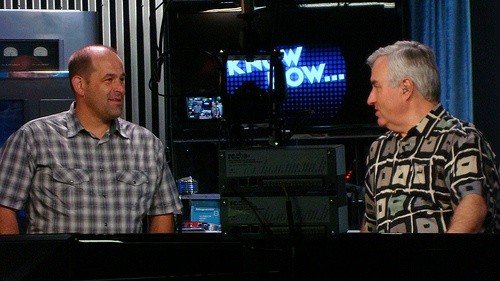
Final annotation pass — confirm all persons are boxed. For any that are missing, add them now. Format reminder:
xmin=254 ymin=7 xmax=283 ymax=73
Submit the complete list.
xmin=361 ymin=41 xmax=500 ymax=233
xmin=0 ymin=45 xmax=184 ymax=234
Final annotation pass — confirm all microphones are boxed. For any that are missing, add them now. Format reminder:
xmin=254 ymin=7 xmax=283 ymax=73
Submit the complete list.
xmin=228 ymin=183 xmax=272 ymax=236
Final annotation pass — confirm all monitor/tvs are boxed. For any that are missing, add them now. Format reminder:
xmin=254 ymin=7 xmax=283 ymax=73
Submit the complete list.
xmin=185 ymin=94 xmax=224 ymax=120
